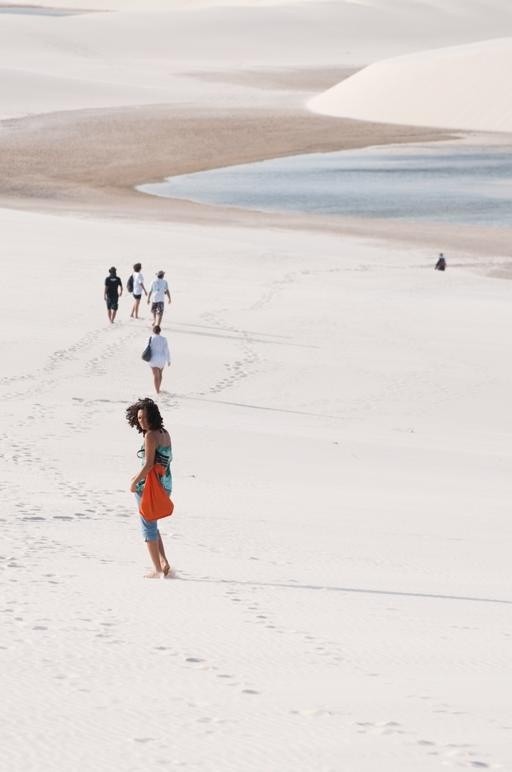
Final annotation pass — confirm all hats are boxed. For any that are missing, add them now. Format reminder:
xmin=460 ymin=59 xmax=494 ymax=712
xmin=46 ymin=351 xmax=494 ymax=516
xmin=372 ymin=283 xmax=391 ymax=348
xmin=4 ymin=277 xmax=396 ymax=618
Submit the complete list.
xmin=109 ymin=267 xmax=116 ymax=272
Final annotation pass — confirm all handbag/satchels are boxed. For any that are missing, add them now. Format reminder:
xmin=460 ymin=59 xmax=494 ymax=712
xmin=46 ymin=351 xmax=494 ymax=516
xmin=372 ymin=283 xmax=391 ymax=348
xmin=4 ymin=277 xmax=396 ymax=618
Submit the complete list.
xmin=141 ymin=336 xmax=152 ymax=361
xmin=126 ymin=274 xmax=133 ymax=292
xmin=138 ymin=462 xmax=175 ymax=521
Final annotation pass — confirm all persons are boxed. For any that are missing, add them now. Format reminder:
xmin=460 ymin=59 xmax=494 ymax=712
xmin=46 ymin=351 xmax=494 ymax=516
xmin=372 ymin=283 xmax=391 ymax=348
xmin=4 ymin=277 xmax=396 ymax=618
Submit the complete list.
xmin=103 ymin=265 xmax=123 ymax=324
xmin=433 ymin=251 xmax=448 ymax=272
xmin=124 ymin=396 xmax=175 ymax=579
xmin=126 ymin=262 xmax=148 ymax=319
xmin=141 ymin=325 xmax=171 ymax=394
xmin=146 ymin=270 xmax=171 ymax=327
